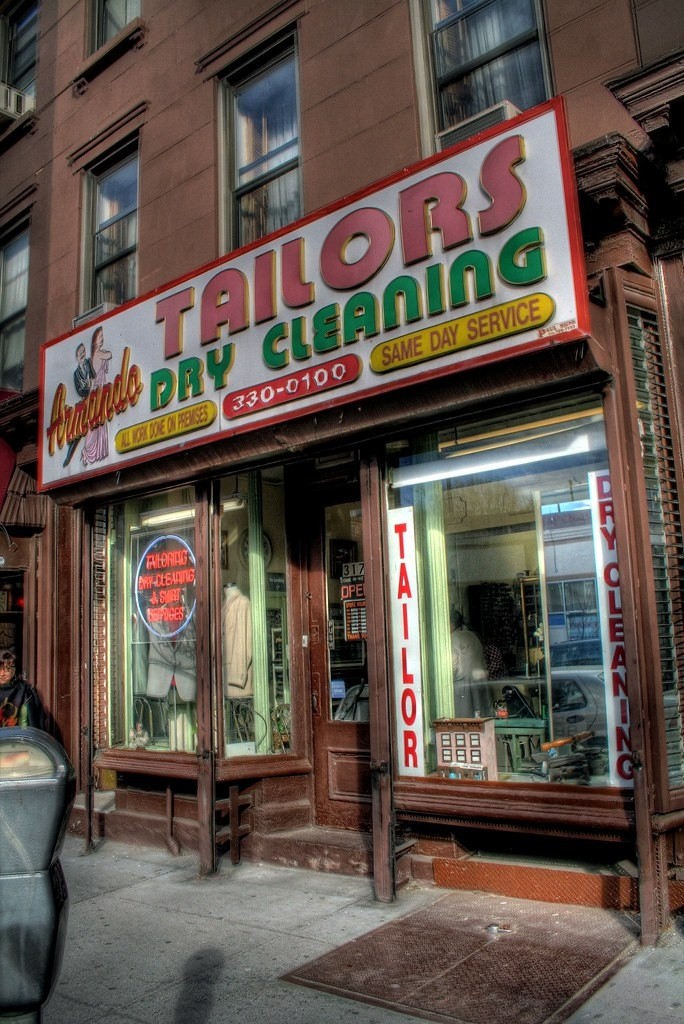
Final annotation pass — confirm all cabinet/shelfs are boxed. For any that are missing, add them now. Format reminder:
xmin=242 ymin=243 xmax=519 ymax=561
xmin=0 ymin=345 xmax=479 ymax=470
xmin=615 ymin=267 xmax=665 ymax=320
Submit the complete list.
xmin=531 ymin=481 xmax=612 ymax=787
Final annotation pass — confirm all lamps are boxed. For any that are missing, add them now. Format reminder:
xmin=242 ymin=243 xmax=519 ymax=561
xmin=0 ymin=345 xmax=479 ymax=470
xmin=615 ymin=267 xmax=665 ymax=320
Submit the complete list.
xmin=139 ymin=475 xmax=247 ymax=526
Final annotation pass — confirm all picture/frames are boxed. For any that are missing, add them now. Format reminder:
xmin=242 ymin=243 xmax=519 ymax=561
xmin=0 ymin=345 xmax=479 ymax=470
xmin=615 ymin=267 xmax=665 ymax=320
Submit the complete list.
xmin=190 ymin=528 xmax=229 ymax=570
xmin=329 ymin=537 xmax=358 ymax=579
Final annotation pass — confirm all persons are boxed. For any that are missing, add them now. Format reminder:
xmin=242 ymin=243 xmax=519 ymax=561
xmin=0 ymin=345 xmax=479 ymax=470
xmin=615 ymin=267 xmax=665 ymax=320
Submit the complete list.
xmin=132 ymin=722 xmax=150 ymax=746
xmin=1 ymin=653 xmax=44 ymax=728
xmin=146 ymin=583 xmax=253 ymax=703
xmin=450 ymin=605 xmax=496 ymax=718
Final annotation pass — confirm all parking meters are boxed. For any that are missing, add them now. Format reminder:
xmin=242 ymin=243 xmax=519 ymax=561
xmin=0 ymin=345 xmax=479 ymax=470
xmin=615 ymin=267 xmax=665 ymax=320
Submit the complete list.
xmin=0 ymin=722 xmax=78 ymax=1023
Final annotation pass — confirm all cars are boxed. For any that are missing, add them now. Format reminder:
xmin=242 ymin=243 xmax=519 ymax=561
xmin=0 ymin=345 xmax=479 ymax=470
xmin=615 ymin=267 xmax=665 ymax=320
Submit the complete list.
xmin=457 ymin=634 xmax=684 ymax=791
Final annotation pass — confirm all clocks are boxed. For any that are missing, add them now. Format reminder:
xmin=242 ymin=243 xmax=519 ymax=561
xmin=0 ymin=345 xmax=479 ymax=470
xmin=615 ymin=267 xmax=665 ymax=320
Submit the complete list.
xmin=237 ymin=527 xmax=273 ymax=572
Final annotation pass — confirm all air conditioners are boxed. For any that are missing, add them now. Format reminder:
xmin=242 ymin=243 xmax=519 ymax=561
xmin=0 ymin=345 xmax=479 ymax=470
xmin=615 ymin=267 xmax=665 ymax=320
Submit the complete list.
xmin=0 ymin=81 xmax=26 ymax=134
xmin=434 ymin=99 xmax=523 ymax=154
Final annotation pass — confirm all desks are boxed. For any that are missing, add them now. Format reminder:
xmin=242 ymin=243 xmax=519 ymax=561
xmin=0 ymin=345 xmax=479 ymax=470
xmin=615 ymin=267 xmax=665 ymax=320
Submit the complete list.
xmin=492 ymin=717 xmax=549 ymax=776
